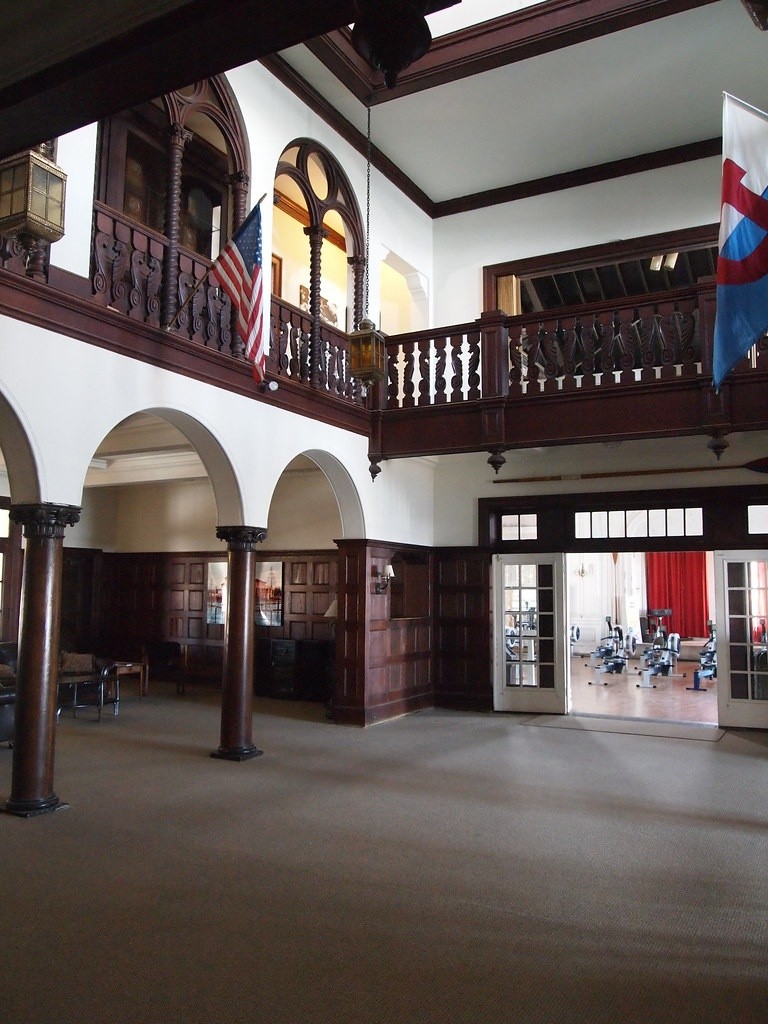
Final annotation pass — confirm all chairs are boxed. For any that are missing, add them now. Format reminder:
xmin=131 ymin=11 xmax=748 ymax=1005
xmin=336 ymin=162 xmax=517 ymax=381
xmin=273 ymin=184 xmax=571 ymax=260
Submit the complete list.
xmin=0 ymin=640 xmax=151 ymax=744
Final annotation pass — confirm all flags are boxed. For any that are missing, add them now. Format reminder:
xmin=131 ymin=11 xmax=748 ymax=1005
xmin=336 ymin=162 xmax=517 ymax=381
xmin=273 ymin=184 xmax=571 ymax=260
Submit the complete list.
xmin=213 ymin=204 xmax=266 ymax=384
xmin=713 ymin=89 xmax=768 ymax=393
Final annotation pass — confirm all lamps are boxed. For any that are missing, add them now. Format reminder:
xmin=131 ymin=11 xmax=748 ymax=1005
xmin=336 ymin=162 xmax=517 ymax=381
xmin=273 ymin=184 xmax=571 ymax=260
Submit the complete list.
xmin=345 ymin=319 xmax=389 ymax=390
xmin=375 ymin=564 xmax=396 ymax=594
xmin=0 ymin=143 xmax=68 ymax=251
